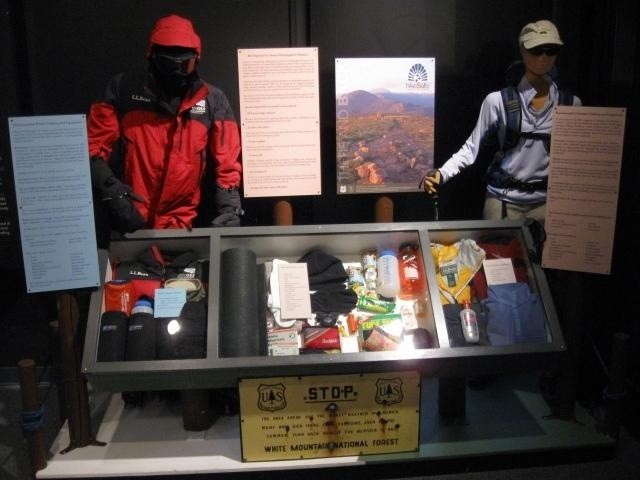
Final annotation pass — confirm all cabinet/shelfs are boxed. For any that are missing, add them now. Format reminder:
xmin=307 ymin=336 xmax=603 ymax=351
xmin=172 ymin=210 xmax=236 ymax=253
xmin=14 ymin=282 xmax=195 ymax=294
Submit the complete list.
xmin=81 ymin=217 xmax=567 ymax=393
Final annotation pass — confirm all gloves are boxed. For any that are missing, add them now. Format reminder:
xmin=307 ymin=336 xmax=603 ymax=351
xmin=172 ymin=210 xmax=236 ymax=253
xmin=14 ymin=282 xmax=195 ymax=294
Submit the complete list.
xmin=209 ymin=189 xmax=245 ymax=226
xmin=86 ymin=157 xmax=146 ymax=233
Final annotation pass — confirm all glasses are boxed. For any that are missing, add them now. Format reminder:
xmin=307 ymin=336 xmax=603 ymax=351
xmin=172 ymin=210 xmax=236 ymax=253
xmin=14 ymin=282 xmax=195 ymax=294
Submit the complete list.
xmin=528 ymin=44 xmax=558 ymax=58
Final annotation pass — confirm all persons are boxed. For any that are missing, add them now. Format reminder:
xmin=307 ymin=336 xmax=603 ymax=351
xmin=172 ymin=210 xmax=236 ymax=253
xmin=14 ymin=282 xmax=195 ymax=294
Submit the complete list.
xmin=87 ymin=16 xmax=245 ymax=232
xmin=418 ymin=19 xmax=583 ymax=265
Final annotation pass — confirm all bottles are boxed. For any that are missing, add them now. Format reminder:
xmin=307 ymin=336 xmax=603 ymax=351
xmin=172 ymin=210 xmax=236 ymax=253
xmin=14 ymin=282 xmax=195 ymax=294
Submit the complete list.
xmin=460 ymin=299 xmax=480 ymax=343
xmin=378 ymin=250 xmax=400 ymax=299
xmin=130 ymin=301 xmax=153 ymax=315
xmin=398 ymin=242 xmax=425 ymax=300
xmin=104 ymin=280 xmax=136 ymax=317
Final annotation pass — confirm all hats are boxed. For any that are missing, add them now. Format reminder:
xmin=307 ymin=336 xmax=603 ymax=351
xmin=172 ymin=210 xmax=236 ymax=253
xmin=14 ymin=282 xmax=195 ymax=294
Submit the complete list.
xmin=518 ymin=20 xmax=564 ymax=50
xmin=147 ymin=15 xmax=201 ymax=56
